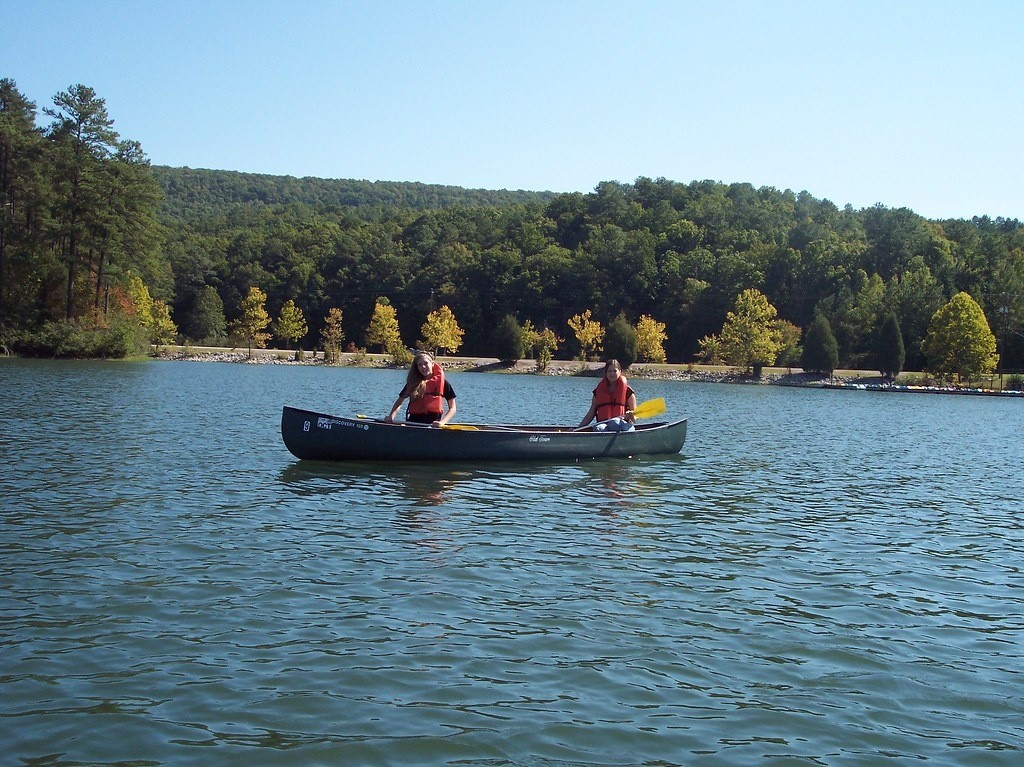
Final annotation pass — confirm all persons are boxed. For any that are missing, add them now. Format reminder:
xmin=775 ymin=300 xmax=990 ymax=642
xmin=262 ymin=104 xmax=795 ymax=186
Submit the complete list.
xmin=385 ymin=349 xmax=457 ymax=427
xmin=564 ymin=359 xmax=638 ymax=433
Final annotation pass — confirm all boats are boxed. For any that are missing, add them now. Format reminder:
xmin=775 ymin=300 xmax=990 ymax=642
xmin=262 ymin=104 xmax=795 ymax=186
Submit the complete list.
xmin=280 ymin=405 xmax=688 ymax=464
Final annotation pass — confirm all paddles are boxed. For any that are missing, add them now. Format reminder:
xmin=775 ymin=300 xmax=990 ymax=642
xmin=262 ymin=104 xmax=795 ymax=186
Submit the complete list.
xmin=356 ymin=414 xmax=479 ymax=431
xmin=559 ymin=398 xmax=666 ymax=432
